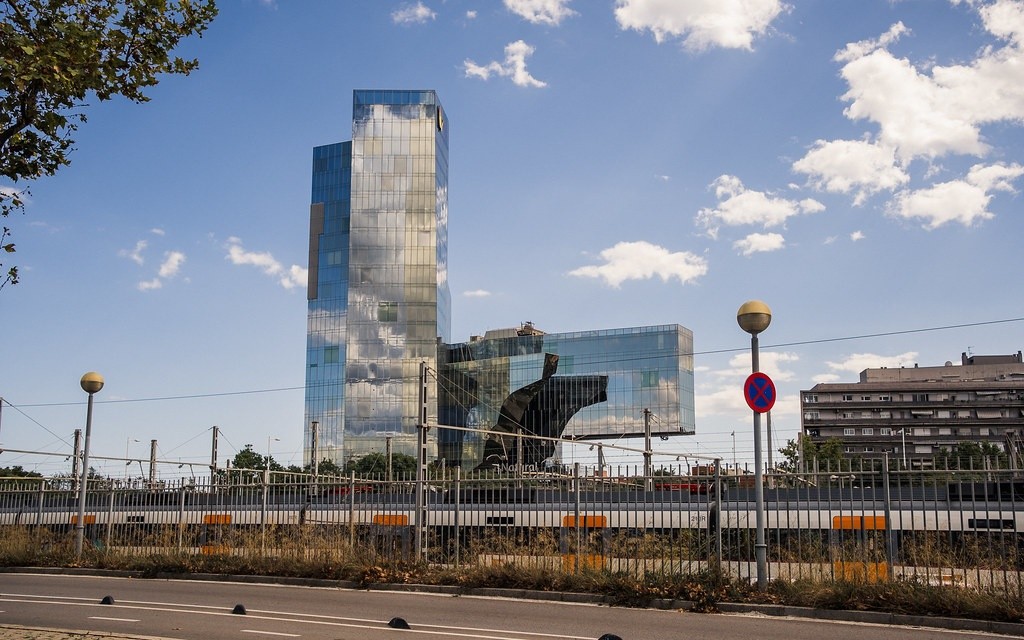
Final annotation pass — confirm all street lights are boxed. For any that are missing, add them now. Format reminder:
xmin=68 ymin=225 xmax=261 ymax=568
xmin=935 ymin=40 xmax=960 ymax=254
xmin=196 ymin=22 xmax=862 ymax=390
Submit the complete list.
xmin=736 ymin=301 xmax=773 ymax=594
xmin=898 ymin=428 xmax=907 ymax=470
xmin=267 ymin=435 xmax=281 ymax=472
xmin=124 ymin=437 xmax=140 ymax=487
xmin=71 ymin=371 xmax=105 ymax=567
xmin=571 ymin=433 xmax=576 ymax=470
xmin=730 ymin=431 xmax=735 ymax=475
